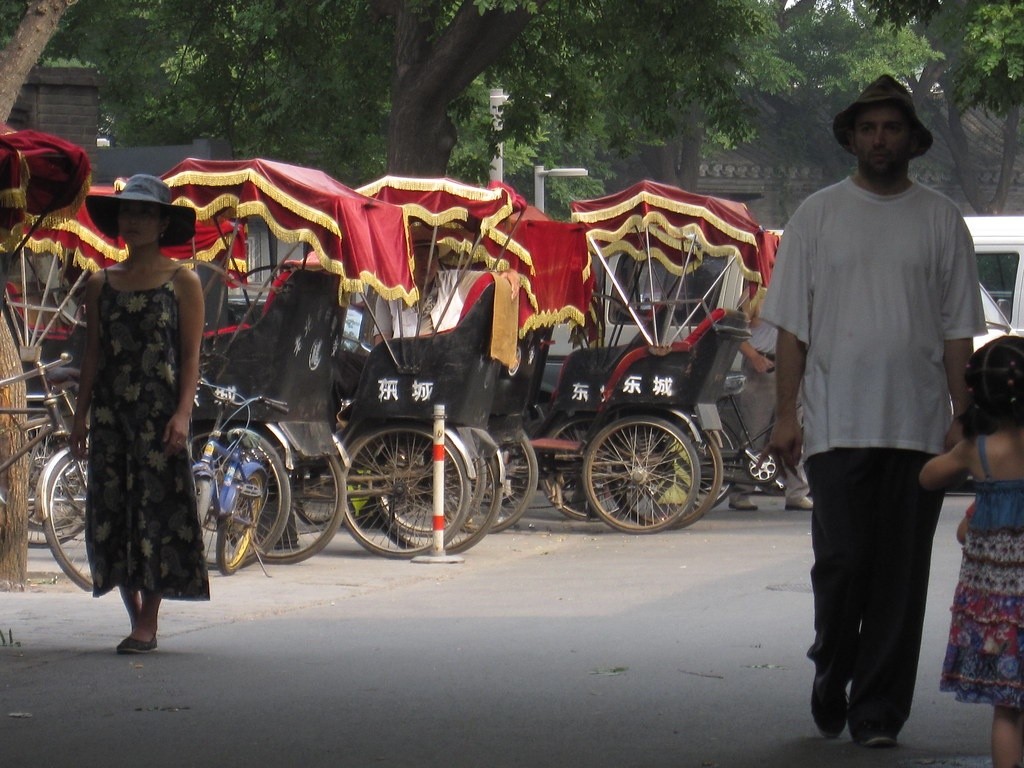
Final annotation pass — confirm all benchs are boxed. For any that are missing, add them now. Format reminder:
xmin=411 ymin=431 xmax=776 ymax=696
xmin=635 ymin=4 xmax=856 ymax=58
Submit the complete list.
xmin=0 ymin=245 xmax=229 ymax=399
xmin=200 ymin=249 xmax=346 ymax=424
xmin=548 ymin=307 xmax=752 ymax=421
xmin=348 ymin=270 xmax=520 ymax=431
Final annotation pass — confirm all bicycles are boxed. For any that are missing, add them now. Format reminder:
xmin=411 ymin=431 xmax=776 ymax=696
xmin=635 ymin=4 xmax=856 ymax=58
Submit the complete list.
xmin=716 ymin=365 xmax=805 ymax=505
xmin=1 ymin=352 xmax=292 ymax=593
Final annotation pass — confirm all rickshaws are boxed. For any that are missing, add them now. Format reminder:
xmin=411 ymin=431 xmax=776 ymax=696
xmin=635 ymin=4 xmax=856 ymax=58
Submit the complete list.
xmin=0 ymin=124 xmax=789 ymax=565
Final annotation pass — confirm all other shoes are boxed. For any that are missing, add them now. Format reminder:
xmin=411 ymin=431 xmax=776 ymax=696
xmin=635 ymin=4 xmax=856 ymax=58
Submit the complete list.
xmin=117 ymin=633 xmax=158 ymax=653
xmin=729 ymin=498 xmax=757 ymax=510
xmin=812 ymin=663 xmax=898 ymax=748
xmin=785 ymin=496 xmax=813 ymax=510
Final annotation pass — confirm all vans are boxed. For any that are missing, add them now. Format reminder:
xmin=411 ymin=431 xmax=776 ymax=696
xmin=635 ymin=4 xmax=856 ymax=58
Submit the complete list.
xmin=962 ymin=215 xmax=1024 ymax=340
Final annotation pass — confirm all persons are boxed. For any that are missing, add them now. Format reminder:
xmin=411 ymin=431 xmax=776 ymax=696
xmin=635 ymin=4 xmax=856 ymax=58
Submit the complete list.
xmin=373 ymin=230 xmax=521 ymax=349
xmin=71 ymin=174 xmax=211 ymax=654
xmin=728 ymin=321 xmax=813 ymax=511
xmin=920 ymin=336 xmax=1024 ymax=768
xmin=758 ymin=76 xmax=989 ymax=744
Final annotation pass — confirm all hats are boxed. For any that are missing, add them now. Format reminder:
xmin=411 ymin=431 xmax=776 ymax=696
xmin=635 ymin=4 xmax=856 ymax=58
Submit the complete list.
xmin=86 ymin=174 xmax=196 ymax=246
xmin=833 ymin=75 xmax=933 ymax=158
xmin=411 ymin=225 xmax=436 ymax=247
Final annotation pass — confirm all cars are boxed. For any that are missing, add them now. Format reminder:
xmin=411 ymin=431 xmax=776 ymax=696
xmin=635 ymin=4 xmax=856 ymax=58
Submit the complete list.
xmin=971 ymin=283 xmax=1024 ymax=355
